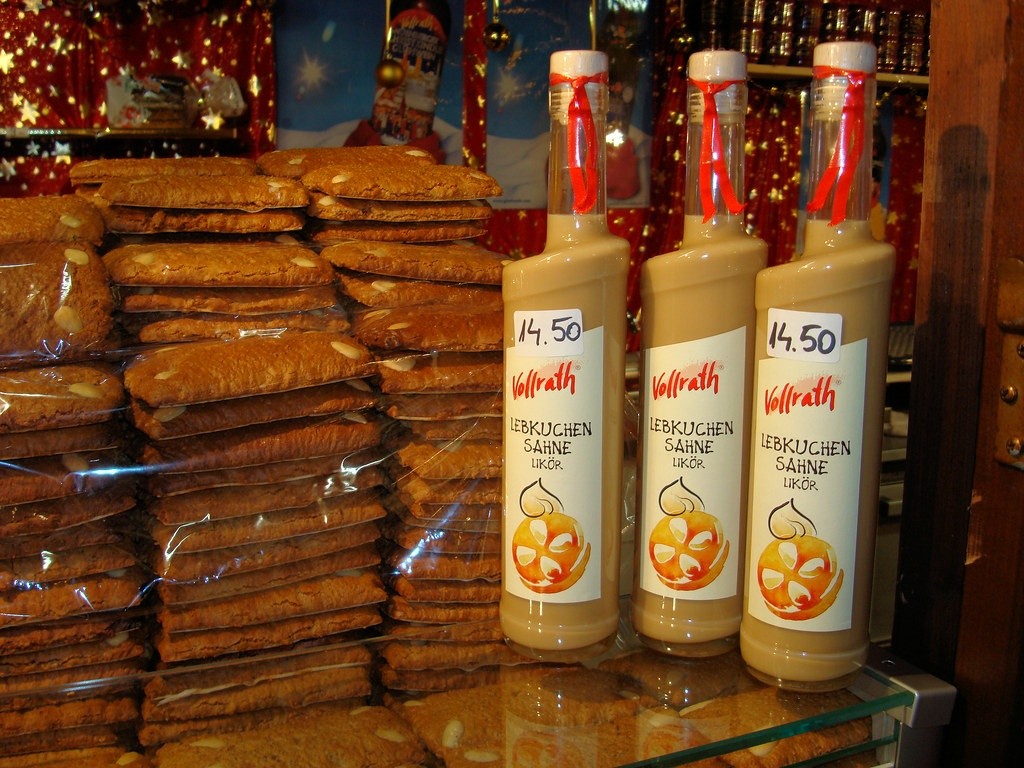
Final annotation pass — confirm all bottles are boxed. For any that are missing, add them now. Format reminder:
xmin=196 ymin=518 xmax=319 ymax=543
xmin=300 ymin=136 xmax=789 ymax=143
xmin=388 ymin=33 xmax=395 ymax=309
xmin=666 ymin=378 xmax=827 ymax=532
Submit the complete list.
xmin=740 ymin=41 xmax=894 ymax=694
xmin=499 ymin=49 xmax=630 ymax=662
xmin=633 ymin=51 xmax=768 ymax=658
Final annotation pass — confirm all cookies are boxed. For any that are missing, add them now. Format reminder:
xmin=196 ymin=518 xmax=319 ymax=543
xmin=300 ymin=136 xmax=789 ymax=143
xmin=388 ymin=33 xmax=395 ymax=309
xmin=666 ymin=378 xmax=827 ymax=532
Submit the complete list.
xmin=0 ymin=147 xmax=874 ymax=768
